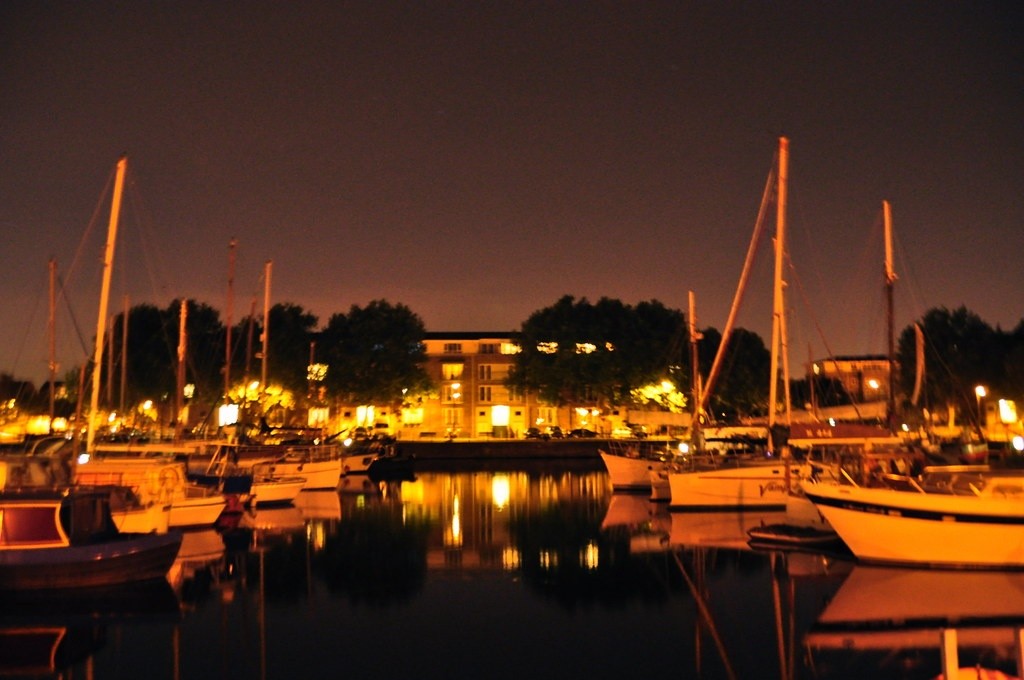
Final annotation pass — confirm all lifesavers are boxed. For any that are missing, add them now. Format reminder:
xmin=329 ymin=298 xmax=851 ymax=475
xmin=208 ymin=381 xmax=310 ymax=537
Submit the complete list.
xmin=160 ymin=467 xmax=179 ymax=490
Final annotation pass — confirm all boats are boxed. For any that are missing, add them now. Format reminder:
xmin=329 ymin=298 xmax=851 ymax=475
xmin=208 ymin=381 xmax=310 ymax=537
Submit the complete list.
xmin=601 ymin=488 xmax=652 ymax=531
xmin=800 ymin=562 xmax=1024 ymax=680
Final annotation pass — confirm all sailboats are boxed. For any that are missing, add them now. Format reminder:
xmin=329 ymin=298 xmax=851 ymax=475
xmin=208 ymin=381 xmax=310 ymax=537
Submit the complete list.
xmin=597 ymin=136 xmax=1023 ymax=572
xmin=0 ymin=149 xmax=399 ymax=601
xmin=668 ymin=507 xmax=798 ymax=680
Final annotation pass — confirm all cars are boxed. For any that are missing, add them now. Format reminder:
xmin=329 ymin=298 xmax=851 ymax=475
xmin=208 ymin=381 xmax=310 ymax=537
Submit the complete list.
xmin=568 ymin=428 xmax=596 ymax=439
xmin=353 ymin=426 xmax=369 ymax=441
xmin=610 ymin=426 xmax=647 ymax=439
xmin=373 ymin=420 xmax=394 ymax=439
xmin=525 ymin=427 xmax=541 ymax=439
xmin=545 ymin=426 xmax=565 ymax=438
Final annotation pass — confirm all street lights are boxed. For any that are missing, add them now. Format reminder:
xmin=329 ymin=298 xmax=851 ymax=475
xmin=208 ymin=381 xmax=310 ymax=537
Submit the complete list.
xmin=976 ymin=384 xmax=985 ymax=425
